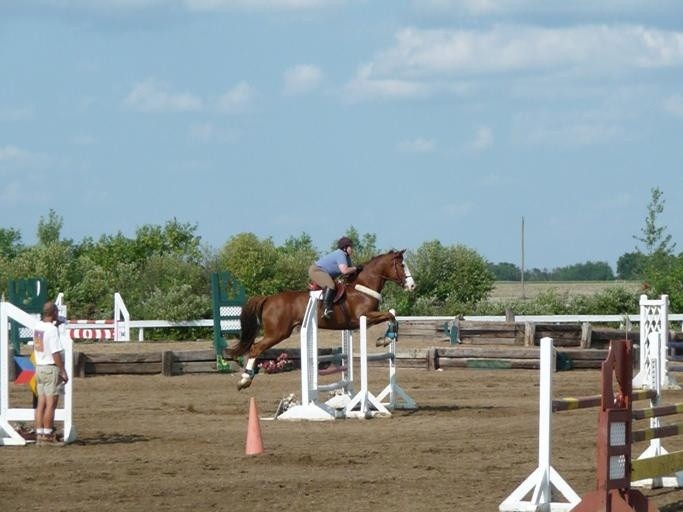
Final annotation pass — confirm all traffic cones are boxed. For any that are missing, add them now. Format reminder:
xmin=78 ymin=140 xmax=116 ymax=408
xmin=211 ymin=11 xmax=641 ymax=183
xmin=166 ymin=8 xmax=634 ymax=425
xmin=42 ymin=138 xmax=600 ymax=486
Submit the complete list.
xmin=246 ymin=397 xmax=264 ymax=455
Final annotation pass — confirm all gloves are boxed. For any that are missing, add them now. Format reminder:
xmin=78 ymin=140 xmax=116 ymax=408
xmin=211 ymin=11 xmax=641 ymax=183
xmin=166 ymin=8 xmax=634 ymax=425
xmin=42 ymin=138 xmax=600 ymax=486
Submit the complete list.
xmin=356 ymin=266 xmax=363 ymax=271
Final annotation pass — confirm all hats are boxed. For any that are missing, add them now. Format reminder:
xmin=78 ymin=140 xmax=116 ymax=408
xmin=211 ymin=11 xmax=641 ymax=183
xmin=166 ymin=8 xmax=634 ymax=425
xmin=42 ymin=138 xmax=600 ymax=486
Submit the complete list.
xmin=44 ymin=302 xmax=58 ymax=312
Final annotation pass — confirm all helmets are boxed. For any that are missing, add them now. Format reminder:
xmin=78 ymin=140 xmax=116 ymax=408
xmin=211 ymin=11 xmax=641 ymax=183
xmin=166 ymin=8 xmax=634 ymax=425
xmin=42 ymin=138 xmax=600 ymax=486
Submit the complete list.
xmin=338 ymin=238 xmax=352 ymax=249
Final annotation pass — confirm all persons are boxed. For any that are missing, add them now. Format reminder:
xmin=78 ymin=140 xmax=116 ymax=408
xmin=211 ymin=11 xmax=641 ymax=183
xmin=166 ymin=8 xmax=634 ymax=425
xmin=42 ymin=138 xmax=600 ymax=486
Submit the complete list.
xmin=32 ymin=302 xmax=69 ymax=446
xmin=309 ymin=237 xmax=362 ymax=319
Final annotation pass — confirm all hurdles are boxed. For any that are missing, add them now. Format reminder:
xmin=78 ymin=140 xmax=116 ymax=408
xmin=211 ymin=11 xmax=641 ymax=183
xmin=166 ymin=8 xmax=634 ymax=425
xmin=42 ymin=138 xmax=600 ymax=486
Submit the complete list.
xmin=336 ymin=309 xmax=418 ymax=418
xmin=274 ymin=297 xmax=360 ymax=421
xmin=498 ymin=337 xmax=683 ymax=512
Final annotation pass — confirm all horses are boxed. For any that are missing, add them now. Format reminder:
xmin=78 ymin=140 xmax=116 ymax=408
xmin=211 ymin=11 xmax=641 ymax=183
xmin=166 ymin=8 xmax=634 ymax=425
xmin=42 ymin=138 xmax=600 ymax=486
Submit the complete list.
xmin=226 ymin=247 xmax=417 ymax=391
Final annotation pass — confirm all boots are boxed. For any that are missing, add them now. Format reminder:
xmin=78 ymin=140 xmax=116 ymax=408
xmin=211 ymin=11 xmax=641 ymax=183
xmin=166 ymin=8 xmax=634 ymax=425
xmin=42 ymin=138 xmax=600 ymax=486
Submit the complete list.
xmin=321 ymin=285 xmax=336 ymax=320
xmin=36 ymin=428 xmax=66 ymax=446
